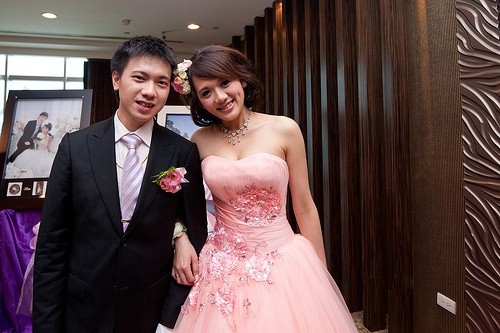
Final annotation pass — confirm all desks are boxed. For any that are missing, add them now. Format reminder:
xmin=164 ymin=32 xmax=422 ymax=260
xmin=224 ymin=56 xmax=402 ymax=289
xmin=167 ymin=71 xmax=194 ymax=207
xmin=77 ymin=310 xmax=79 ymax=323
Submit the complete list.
xmin=0 ymin=209 xmax=42 ymax=333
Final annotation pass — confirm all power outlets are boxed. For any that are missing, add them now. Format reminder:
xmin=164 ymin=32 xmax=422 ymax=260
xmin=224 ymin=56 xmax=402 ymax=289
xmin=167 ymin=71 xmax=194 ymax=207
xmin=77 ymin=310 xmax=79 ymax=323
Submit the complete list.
xmin=436 ymin=292 xmax=456 ymax=315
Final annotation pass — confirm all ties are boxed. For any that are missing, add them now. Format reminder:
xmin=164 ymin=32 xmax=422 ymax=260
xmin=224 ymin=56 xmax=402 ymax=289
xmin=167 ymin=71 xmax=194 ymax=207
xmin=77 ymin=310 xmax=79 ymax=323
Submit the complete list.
xmin=120 ymin=134 xmax=145 ymax=231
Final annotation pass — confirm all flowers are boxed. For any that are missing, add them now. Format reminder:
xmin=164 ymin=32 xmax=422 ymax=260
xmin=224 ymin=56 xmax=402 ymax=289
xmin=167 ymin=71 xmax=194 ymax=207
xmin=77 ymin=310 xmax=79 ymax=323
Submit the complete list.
xmin=150 ymin=165 xmax=190 ymax=194
xmin=170 ymin=58 xmax=193 ymax=94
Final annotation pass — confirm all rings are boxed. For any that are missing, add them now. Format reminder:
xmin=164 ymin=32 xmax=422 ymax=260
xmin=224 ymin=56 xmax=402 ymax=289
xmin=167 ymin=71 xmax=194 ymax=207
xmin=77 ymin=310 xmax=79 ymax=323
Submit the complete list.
xmin=175 ymin=274 xmax=180 ymax=278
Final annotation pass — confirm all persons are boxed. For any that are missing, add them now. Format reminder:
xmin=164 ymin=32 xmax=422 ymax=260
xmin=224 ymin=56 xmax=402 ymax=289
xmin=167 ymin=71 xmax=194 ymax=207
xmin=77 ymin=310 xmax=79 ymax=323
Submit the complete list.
xmin=171 ymin=45 xmax=359 ymax=333
xmin=6 ymin=112 xmax=56 ymax=178
xmin=33 ymin=35 xmax=209 ymax=333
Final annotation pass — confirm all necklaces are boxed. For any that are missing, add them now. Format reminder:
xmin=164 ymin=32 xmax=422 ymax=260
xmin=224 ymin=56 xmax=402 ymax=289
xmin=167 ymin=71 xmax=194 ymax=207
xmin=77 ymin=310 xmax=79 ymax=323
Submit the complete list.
xmin=219 ymin=107 xmax=252 ymax=146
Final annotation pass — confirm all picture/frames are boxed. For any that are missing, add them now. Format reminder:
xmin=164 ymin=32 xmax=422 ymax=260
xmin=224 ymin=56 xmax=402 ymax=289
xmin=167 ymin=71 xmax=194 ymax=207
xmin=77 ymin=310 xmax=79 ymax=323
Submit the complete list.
xmin=156 ymin=106 xmax=211 ymax=141
xmin=0 ymin=89 xmax=93 ymax=212
